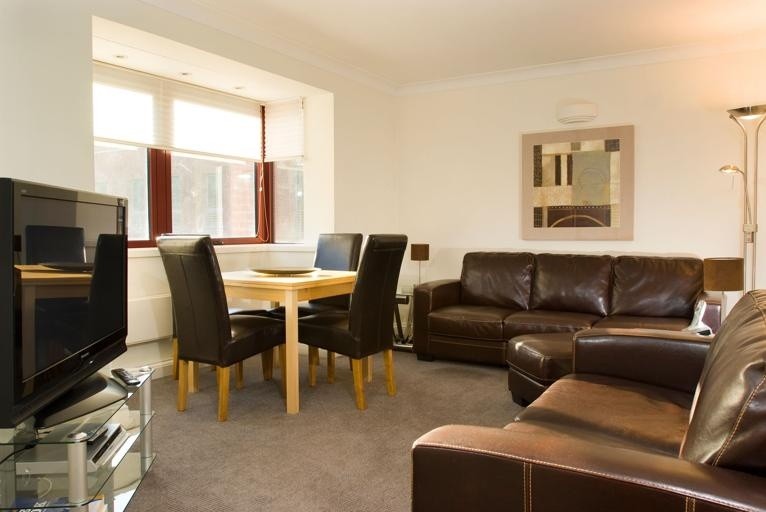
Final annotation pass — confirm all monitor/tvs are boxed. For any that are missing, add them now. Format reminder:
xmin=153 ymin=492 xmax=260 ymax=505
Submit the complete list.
xmin=0 ymin=177 xmax=128 ymax=429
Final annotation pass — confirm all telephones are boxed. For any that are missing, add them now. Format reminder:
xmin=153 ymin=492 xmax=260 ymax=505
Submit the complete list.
xmin=681 ymin=300 xmax=713 ymax=337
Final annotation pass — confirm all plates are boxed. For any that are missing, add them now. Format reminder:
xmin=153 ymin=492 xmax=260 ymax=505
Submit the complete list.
xmin=42 ymin=261 xmax=94 ymax=273
xmin=251 ymin=268 xmax=321 ymax=277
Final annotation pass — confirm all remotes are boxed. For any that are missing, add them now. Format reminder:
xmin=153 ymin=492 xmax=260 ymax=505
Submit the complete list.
xmin=112 ymin=368 xmax=141 ymax=386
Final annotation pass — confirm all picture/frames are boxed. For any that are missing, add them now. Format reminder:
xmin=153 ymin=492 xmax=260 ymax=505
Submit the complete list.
xmin=521 ymin=125 xmax=635 ymax=240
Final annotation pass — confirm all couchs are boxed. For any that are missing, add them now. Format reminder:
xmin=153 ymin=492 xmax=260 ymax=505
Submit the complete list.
xmin=412 ymin=289 xmax=766 ymax=512
xmin=413 ymin=252 xmax=722 ymax=365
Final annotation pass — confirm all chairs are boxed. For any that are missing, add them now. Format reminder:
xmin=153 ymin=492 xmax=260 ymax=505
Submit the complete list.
xmin=271 ymin=233 xmax=363 ymax=384
xmin=155 ymin=236 xmax=286 ymax=421
xmin=161 ymin=233 xmax=268 ymax=379
xmin=298 ymin=234 xmax=407 ymax=410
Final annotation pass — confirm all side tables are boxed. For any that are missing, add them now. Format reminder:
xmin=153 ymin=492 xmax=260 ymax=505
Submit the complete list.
xmin=392 ymin=286 xmax=414 ymax=353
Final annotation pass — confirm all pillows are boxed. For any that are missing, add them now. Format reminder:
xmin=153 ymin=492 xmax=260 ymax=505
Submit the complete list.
xmin=459 ymin=252 xmax=703 ymax=315
xmin=679 ymin=288 xmax=766 ymax=469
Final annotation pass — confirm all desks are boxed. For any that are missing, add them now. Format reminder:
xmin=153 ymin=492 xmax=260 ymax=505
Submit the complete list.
xmin=187 ymin=270 xmax=372 ymax=414
xmin=0 ymin=367 xmax=158 ymax=512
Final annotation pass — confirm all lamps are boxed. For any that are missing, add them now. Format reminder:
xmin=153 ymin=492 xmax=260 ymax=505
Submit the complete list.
xmin=718 ymin=104 xmax=766 ymax=294
xmin=703 ymin=257 xmax=744 ymax=325
xmin=411 ymin=244 xmax=429 ymax=285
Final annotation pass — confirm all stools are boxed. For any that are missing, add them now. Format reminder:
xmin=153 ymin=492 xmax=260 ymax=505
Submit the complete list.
xmin=506 ymin=333 xmax=574 ymax=406
